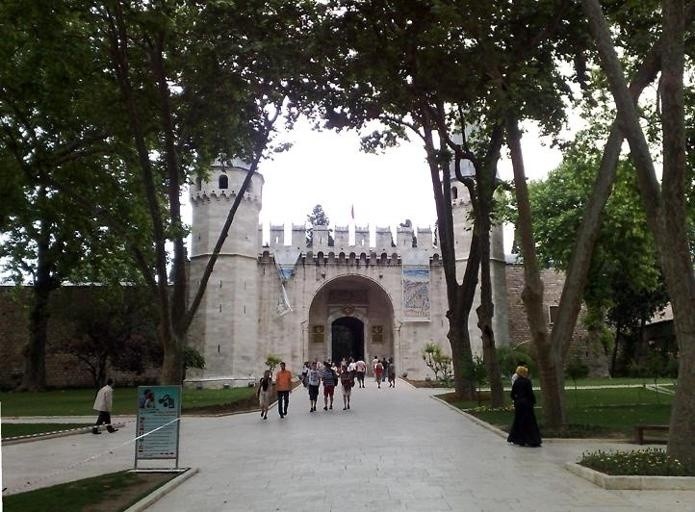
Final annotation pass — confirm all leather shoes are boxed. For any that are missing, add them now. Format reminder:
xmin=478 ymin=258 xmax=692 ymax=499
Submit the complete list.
xmin=261 ymin=413 xmax=266 ymax=419
xmin=390 ymin=384 xmax=395 ymax=387
xmin=359 ymin=385 xmax=365 ymax=388
xmin=310 ymin=405 xmax=350 ymax=412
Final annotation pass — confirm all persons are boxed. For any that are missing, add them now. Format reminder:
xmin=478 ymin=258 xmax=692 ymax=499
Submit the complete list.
xmin=256 ymin=370 xmax=273 ymax=420
xmin=507 ymin=367 xmax=543 ymax=448
xmin=145 ymin=393 xmax=154 ymax=408
xmin=139 ymin=389 xmax=151 ymax=407
xmin=92 ymin=378 xmax=115 ymax=434
xmin=373 ymin=356 xmax=395 ymax=389
xmin=301 ymin=356 xmax=367 ymax=413
xmin=512 ymin=361 xmax=528 ymax=384
xmin=275 ymin=362 xmax=293 ymax=418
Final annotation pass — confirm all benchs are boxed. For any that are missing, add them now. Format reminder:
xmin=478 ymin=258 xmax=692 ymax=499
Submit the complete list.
xmin=630 ymin=421 xmax=673 ymax=447
